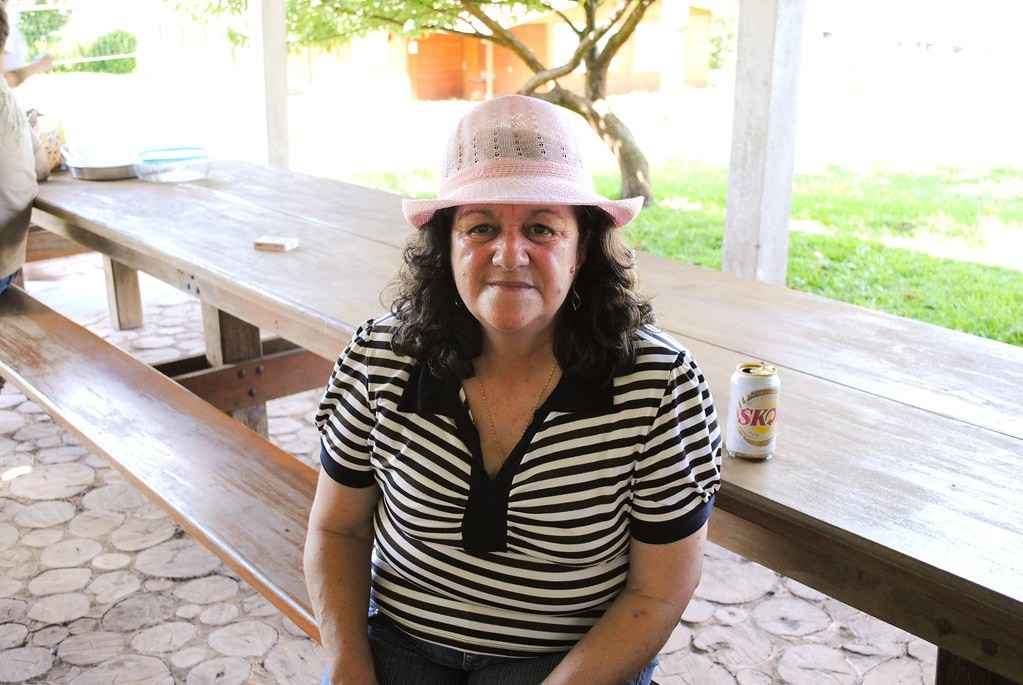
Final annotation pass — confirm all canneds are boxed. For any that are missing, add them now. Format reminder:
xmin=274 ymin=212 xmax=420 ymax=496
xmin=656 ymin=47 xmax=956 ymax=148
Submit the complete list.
xmin=725 ymin=361 xmax=780 ymax=460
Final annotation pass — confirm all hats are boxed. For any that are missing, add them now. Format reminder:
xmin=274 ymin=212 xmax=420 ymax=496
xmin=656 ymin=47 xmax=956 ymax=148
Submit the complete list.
xmin=400 ymin=95 xmax=644 ymax=230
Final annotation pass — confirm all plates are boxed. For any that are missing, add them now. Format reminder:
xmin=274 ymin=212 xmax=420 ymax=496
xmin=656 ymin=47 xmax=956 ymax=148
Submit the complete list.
xmin=62 ymin=163 xmax=137 ymax=180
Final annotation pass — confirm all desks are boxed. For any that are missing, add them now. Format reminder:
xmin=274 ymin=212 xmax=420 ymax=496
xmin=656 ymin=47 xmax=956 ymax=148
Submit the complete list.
xmin=34 ymin=157 xmax=1023 ymax=685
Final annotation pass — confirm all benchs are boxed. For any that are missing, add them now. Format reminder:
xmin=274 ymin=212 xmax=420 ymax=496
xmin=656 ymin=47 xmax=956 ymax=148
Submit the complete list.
xmin=0 ymin=279 xmax=660 ymax=685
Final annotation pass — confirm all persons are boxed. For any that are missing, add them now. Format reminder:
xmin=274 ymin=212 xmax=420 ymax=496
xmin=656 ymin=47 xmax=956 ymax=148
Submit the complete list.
xmin=0 ymin=0 xmax=55 ymax=293
xmin=303 ymin=94 xmax=722 ymax=685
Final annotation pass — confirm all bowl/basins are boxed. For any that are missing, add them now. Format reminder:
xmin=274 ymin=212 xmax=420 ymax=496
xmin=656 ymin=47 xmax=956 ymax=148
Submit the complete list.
xmin=135 ymin=147 xmax=211 ymax=183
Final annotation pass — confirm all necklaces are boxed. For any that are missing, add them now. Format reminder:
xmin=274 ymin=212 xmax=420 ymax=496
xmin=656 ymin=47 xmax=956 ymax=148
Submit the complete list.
xmin=476 ymin=358 xmax=557 ymax=465
